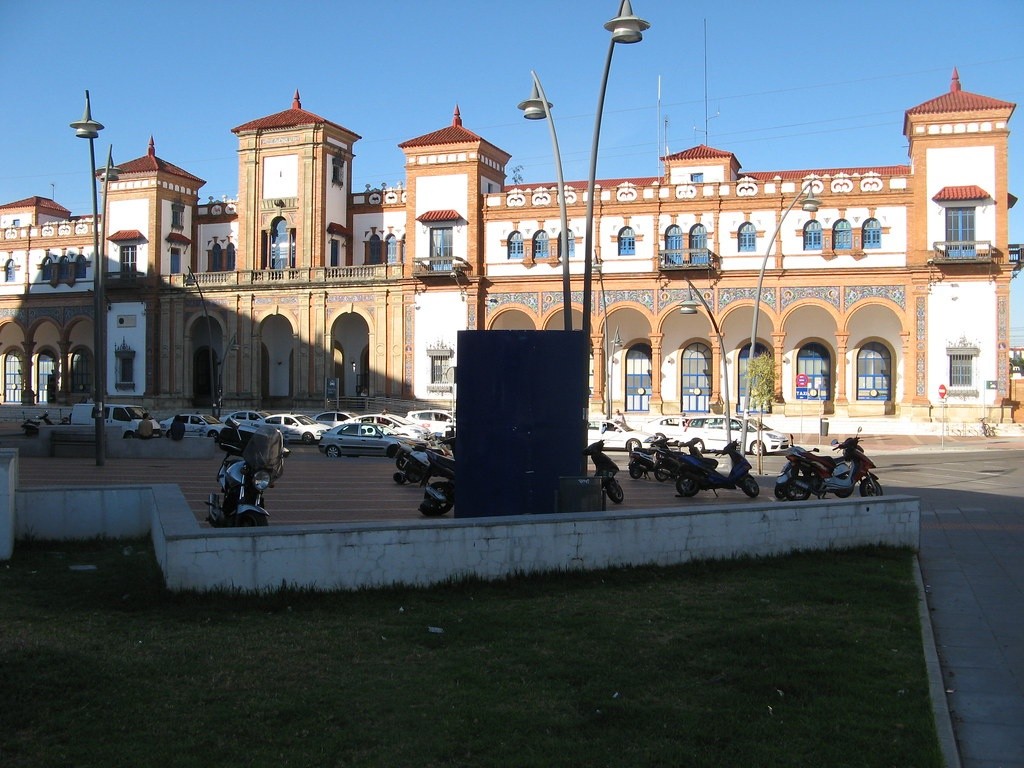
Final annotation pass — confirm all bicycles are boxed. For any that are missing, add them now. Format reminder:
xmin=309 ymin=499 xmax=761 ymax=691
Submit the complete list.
xmin=978 ymin=418 xmax=990 ymax=437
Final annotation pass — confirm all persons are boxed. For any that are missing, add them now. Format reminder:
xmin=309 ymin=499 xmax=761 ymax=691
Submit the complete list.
xmin=138 ymin=413 xmax=153 ymax=439
xmin=614 ymin=409 xmax=626 ymax=425
xmin=171 ymin=414 xmax=186 ymax=441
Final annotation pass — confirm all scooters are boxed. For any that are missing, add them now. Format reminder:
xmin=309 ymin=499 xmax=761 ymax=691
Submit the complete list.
xmin=393 ymin=427 xmax=456 ymax=515
xmin=582 ymin=437 xmax=624 ymax=503
xmin=627 ymin=436 xmax=759 ymax=498
xmin=22 ymin=408 xmax=72 ymax=438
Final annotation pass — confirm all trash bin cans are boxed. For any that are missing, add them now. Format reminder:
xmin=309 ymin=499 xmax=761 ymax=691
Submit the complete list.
xmin=820 ymin=418 xmax=829 ymax=437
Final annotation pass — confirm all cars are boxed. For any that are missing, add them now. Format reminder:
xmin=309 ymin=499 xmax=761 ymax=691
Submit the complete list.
xmin=318 ymin=423 xmax=411 ymax=458
xmin=158 ymin=413 xmax=229 ymax=439
xmin=641 ymin=415 xmax=690 ymax=443
xmin=587 ymin=419 xmax=656 ymax=450
xmin=221 ymin=409 xmax=456 ymax=444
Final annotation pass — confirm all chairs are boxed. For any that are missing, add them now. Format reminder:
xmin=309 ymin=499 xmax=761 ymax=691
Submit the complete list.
xmin=711 ymin=419 xmax=739 ymax=430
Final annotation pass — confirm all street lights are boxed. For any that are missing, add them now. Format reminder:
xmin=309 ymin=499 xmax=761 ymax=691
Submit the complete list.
xmin=183 ymin=269 xmax=246 ymax=419
xmin=658 ymin=179 xmax=822 ymax=455
xmin=593 ymin=250 xmax=625 ymax=421
xmin=69 ymin=88 xmax=119 ymax=462
xmin=515 ymin=0 xmax=650 ymax=479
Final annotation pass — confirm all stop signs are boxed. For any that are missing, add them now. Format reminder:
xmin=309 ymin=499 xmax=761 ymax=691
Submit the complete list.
xmin=938 ymin=385 xmax=947 ymax=398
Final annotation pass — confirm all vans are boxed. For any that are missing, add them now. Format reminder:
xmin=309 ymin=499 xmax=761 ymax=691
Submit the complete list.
xmin=72 ymin=402 xmax=162 ymax=439
xmin=683 ymin=416 xmax=788 ymax=455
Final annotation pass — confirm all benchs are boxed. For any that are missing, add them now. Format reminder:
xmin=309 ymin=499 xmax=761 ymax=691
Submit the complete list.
xmin=50 ymin=431 xmax=108 ymax=458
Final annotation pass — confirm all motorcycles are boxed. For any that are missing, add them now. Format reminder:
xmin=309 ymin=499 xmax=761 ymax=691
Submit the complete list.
xmin=203 ymin=418 xmax=289 ymax=527
xmin=773 ymin=425 xmax=884 ymax=500
